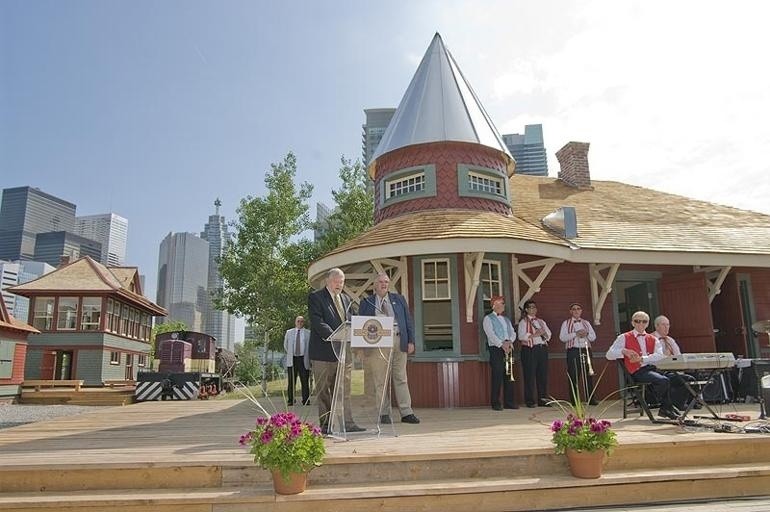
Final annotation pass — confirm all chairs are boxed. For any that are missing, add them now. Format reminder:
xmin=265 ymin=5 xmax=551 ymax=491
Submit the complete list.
xmin=614 ymin=356 xmax=663 ymax=427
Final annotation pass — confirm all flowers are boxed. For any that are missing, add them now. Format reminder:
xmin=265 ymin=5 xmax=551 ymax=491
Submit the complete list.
xmin=220 ymin=361 xmax=346 ymax=485
xmin=535 ymin=353 xmax=644 ymax=455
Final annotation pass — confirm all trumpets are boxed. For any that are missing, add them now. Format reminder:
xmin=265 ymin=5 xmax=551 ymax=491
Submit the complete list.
xmin=505 ymin=345 xmax=516 ymax=382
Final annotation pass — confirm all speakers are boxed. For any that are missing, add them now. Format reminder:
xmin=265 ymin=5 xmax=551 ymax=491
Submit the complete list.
xmin=697 ymin=370 xmax=723 ymax=401
xmin=684 ymin=373 xmax=703 ymax=404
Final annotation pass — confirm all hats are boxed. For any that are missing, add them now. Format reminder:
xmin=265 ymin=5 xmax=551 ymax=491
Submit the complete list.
xmin=489 ymin=295 xmax=506 ymax=308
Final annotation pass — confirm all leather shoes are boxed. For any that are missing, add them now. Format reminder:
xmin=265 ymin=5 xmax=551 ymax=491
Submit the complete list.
xmin=492 ymin=399 xmax=598 ymax=410
xmin=321 ymin=414 xmax=421 ymax=434
xmin=658 ymin=407 xmax=681 ymax=420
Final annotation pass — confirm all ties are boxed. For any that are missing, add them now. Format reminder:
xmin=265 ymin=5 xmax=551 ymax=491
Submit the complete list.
xmin=296 ymin=330 xmax=300 ymax=355
xmin=381 ymin=298 xmax=387 ymax=312
xmin=334 ymin=293 xmax=346 ymax=326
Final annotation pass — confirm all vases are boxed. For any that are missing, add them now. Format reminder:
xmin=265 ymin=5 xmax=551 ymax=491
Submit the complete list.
xmin=272 ymin=464 xmax=307 ymax=495
xmin=565 ymin=447 xmax=605 ymax=480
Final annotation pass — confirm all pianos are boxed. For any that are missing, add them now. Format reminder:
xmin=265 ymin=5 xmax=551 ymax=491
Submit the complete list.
xmin=655 ymin=352 xmax=736 ymax=370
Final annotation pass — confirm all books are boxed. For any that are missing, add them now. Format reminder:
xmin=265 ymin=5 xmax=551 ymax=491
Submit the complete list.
xmin=335 ymin=326 xmax=351 ymax=340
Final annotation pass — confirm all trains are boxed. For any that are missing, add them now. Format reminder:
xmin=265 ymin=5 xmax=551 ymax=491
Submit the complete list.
xmin=135 ymin=329 xmax=244 ymax=401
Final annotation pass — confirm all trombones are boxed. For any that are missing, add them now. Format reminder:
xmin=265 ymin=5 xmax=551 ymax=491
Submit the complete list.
xmin=579 ymin=336 xmax=597 ymax=402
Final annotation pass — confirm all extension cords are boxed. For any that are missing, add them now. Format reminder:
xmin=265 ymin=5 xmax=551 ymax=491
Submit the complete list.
xmin=744 ymin=427 xmax=768 ymax=434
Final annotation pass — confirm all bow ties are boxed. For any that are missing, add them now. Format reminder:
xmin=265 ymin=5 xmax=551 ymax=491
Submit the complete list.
xmin=531 ymin=318 xmax=537 ymax=322
xmin=659 ymin=337 xmax=666 ymax=340
xmin=637 ymin=334 xmax=646 ymax=336
xmin=497 ymin=313 xmax=504 ymax=316
xmin=574 ymin=320 xmax=581 ymax=323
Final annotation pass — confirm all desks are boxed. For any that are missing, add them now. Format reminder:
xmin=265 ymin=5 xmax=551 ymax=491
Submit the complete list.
xmin=633 ymin=366 xmax=737 ymax=423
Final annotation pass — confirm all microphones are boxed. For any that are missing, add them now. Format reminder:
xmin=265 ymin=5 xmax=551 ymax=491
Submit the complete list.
xmin=364 ymin=297 xmax=385 ymax=314
xmin=346 ymin=299 xmax=354 ymax=322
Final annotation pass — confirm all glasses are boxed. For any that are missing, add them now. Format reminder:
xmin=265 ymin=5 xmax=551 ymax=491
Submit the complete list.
xmin=634 ymin=319 xmax=648 ymax=324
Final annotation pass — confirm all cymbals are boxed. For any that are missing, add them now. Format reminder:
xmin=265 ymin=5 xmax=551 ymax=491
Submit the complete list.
xmin=752 ymin=319 xmax=770 ymax=333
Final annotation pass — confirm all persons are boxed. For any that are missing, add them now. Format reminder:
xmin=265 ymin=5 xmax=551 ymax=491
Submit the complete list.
xmin=282 ymin=316 xmax=312 ymax=407
xmin=606 ymin=311 xmax=684 ymax=421
xmin=308 ymin=269 xmax=365 ymax=434
xmin=559 ymin=303 xmax=599 ymax=405
xmin=359 ymin=273 xmax=421 ymax=424
xmin=517 ymin=299 xmax=553 ymax=408
xmin=650 ymin=314 xmax=703 ymax=410
xmin=483 ymin=296 xmax=519 ymax=412
